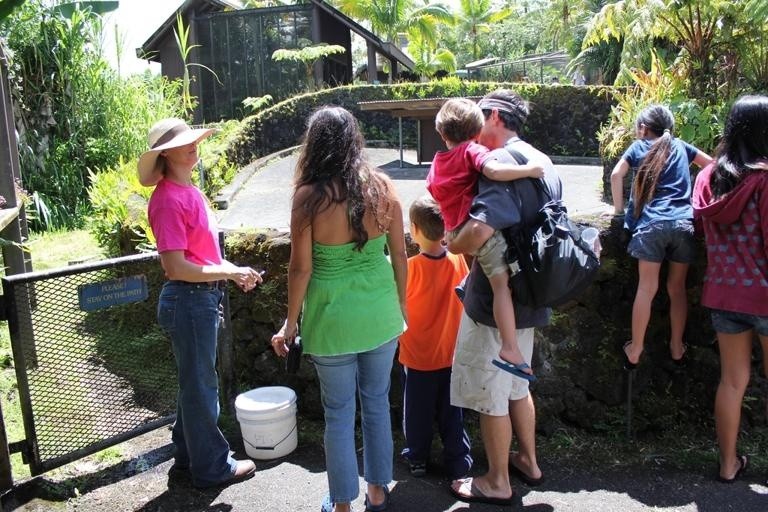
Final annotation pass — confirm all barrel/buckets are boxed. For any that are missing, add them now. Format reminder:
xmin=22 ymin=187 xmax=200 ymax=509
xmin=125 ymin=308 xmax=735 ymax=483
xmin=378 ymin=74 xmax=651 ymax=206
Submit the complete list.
xmin=234 ymin=385 xmax=298 ymax=460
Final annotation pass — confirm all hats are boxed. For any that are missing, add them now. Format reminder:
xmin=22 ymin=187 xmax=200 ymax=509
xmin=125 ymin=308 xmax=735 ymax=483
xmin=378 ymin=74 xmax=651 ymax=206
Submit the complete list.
xmin=136 ymin=117 xmax=217 ymax=188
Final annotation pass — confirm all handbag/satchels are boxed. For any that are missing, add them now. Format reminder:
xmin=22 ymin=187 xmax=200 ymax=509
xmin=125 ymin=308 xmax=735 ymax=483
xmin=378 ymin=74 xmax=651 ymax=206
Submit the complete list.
xmin=499 ymin=201 xmax=603 ymax=310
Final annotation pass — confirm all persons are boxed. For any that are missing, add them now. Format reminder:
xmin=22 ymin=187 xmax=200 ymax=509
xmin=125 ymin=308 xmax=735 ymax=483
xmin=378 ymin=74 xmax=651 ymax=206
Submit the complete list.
xmin=609 ymin=103 xmax=717 ymax=371
xmin=397 ymin=195 xmax=475 ymax=478
xmin=424 ymin=95 xmax=545 ymax=383
xmin=270 ymin=102 xmax=410 ymax=512
xmin=440 ymin=87 xmax=564 ymax=505
xmin=135 ymin=115 xmax=263 ymax=486
xmin=690 ymin=92 xmax=767 ymax=485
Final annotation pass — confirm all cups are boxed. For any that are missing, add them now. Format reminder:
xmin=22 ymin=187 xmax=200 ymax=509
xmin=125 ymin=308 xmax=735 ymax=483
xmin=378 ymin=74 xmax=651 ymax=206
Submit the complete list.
xmin=580 ymin=226 xmax=603 ymax=254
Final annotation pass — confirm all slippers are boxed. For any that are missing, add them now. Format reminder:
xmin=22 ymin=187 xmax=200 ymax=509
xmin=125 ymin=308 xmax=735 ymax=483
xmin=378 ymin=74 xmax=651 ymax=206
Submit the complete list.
xmin=449 ymin=476 xmax=519 ymax=507
xmin=668 ymin=332 xmax=692 ymax=367
xmin=364 ymin=481 xmax=389 ymax=512
xmin=321 ymin=491 xmax=351 ymax=512
xmin=715 ymin=452 xmax=750 ymax=484
xmin=489 ymin=357 xmax=539 ymax=386
xmin=508 ymin=447 xmax=545 ymax=487
xmin=619 ymin=333 xmax=644 ymax=373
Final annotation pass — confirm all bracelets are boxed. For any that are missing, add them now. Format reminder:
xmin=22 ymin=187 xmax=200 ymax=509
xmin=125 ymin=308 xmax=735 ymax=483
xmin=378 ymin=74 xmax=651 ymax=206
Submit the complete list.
xmin=284 ymin=319 xmax=298 ymax=345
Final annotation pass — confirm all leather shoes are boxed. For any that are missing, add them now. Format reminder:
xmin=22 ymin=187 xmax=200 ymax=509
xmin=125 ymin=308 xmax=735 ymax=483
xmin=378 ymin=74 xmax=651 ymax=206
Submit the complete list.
xmin=194 ymin=458 xmax=257 ymax=489
xmin=172 ymin=453 xmax=190 ymax=470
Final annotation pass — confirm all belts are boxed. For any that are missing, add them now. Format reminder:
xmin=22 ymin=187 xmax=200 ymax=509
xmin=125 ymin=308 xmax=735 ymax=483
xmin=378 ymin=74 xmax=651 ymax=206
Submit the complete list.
xmin=182 ymin=277 xmax=231 ymax=290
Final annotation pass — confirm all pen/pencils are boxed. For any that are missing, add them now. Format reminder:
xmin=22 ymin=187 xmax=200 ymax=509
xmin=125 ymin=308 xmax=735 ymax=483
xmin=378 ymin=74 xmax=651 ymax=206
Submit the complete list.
xmin=259 ymin=270 xmax=265 ymax=277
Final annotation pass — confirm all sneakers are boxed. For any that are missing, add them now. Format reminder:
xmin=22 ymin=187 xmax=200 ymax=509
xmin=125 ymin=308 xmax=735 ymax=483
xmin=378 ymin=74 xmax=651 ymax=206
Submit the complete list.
xmin=448 ymin=470 xmax=470 ymax=482
xmin=406 ymin=459 xmax=428 ymax=476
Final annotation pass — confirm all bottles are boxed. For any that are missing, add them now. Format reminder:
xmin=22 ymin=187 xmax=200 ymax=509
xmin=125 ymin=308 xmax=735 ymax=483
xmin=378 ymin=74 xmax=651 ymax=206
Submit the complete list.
xmin=506 ymin=258 xmax=522 ymax=278
